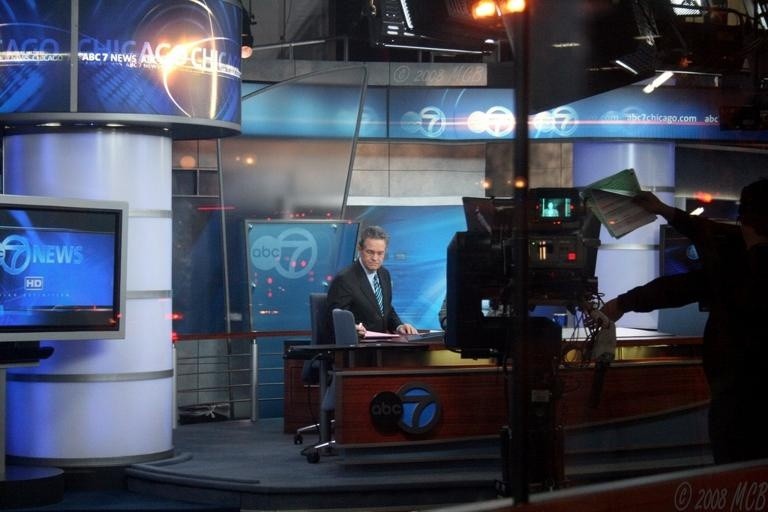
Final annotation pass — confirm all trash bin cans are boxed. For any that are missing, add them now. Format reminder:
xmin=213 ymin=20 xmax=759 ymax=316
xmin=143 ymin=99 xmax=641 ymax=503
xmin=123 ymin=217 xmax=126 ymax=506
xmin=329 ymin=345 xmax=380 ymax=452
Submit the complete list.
xmin=178 ymin=401 xmax=231 ymax=424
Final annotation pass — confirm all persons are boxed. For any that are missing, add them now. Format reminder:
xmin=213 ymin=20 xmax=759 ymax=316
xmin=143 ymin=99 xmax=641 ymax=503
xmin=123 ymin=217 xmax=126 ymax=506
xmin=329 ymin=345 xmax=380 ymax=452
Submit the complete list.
xmin=325 ymin=226 xmax=419 ymax=368
xmin=584 ymin=182 xmax=768 ymax=466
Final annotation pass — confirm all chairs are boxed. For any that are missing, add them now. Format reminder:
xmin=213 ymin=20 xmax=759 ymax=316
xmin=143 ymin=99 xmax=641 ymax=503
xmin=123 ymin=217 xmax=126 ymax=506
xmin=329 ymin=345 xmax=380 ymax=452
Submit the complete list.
xmin=293 ymin=292 xmax=337 ymax=443
xmin=302 ymin=307 xmax=360 ymax=462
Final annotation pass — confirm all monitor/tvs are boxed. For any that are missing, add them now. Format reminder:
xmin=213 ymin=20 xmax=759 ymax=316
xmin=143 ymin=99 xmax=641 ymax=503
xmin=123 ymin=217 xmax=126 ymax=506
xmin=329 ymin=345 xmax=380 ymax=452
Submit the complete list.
xmin=0 ymin=194 xmax=129 ymax=342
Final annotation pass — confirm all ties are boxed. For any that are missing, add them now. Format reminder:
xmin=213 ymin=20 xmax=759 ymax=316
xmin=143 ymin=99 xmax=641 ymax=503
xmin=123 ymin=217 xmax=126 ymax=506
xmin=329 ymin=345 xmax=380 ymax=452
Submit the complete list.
xmin=373 ymin=273 xmax=384 ymax=317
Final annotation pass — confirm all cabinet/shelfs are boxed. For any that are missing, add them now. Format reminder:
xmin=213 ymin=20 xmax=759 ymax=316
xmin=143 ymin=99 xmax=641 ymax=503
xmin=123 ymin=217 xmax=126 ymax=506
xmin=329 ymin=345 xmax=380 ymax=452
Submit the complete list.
xmin=281 ymin=338 xmax=337 ymax=436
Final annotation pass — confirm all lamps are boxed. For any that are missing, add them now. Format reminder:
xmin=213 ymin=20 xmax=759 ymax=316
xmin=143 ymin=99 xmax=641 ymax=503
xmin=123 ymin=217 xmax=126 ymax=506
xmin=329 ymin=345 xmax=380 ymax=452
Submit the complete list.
xmin=238 ymin=0 xmax=257 ymax=59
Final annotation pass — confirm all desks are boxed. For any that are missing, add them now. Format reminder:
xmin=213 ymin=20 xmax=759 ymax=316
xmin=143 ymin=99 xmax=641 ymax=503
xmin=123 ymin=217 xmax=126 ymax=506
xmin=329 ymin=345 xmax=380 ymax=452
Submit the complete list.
xmin=288 ymin=325 xmax=711 ymax=458
